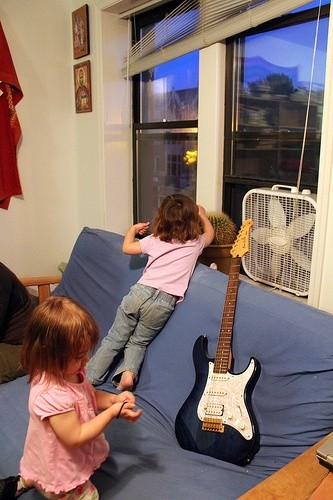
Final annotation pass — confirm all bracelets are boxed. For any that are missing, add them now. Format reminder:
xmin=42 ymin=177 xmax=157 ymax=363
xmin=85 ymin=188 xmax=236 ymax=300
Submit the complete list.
xmin=116 ymin=402 xmax=128 ymax=419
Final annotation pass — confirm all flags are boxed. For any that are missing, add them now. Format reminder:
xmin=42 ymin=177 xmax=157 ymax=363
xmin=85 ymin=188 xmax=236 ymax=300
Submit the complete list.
xmin=0 ymin=22 xmax=24 ymax=210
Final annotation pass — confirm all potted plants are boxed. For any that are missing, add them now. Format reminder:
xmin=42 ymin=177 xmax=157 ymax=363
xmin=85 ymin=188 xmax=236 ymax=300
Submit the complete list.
xmin=198 ymin=211 xmax=237 ymax=275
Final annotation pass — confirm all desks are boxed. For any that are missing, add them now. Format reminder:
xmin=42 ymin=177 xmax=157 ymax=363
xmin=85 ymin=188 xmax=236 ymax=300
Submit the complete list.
xmin=236 ymin=430 xmax=333 ymax=500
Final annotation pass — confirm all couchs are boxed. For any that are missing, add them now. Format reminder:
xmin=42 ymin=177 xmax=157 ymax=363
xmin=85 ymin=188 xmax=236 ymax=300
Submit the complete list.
xmin=0 ymin=227 xmax=333 ymax=500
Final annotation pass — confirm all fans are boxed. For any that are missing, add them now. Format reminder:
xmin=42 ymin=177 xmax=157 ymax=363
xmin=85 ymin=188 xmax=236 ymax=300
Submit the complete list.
xmin=240 ymin=183 xmax=318 ymax=296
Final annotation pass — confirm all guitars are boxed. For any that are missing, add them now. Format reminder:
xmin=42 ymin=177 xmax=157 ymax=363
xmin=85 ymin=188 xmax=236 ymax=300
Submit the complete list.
xmin=174 ymin=218 xmax=262 ymax=468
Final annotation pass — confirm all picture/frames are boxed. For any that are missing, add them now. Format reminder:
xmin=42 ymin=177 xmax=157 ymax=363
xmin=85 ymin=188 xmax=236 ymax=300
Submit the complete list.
xmin=72 ymin=60 xmax=93 ymax=114
xmin=71 ymin=4 xmax=90 ymax=60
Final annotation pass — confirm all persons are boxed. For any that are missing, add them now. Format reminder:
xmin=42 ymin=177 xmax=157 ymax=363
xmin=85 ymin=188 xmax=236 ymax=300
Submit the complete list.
xmin=0 ymin=262 xmax=36 ymax=384
xmin=84 ymin=193 xmax=215 ymax=392
xmin=0 ymin=296 xmax=142 ymax=500
